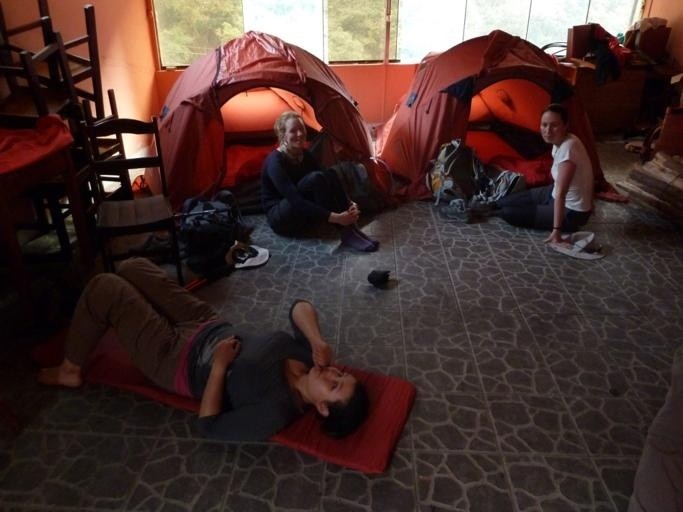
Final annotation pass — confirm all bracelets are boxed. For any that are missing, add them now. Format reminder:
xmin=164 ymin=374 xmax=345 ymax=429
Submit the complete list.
xmin=553 ymin=227 xmax=560 ymax=229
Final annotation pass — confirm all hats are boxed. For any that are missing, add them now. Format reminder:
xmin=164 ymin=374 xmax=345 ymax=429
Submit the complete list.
xmin=550 ymin=231 xmax=605 ymax=260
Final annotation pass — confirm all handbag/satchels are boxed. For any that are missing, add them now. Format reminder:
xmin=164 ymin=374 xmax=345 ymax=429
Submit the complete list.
xmin=624 ymin=25 xmax=672 ymax=56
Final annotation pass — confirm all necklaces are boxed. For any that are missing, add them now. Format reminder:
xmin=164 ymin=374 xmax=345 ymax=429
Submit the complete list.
xmin=287 ymin=148 xmax=301 ymax=165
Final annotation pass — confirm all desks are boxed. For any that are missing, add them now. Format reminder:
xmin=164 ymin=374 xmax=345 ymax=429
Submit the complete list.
xmin=0 ymin=125 xmax=93 ymax=321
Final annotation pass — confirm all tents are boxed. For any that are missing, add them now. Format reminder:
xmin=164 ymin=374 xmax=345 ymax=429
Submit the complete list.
xmin=375 ymin=30 xmax=630 ymax=202
xmin=131 ymin=31 xmax=401 ymax=230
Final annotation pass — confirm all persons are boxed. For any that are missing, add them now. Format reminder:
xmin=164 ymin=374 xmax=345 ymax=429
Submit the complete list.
xmin=260 ymin=111 xmax=380 ymax=252
xmin=500 ymin=103 xmax=595 ymax=246
xmin=37 ymin=256 xmax=369 ymax=442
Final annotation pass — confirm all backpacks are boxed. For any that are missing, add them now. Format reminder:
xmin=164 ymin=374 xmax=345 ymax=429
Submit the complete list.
xmin=328 ymin=158 xmax=370 ymax=200
xmin=178 ymin=190 xmax=254 ymax=244
xmin=425 ymin=137 xmax=489 ymax=206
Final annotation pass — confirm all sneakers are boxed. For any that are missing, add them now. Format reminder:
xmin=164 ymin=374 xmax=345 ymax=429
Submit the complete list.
xmin=438 ymin=198 xmax=471 ymax=221
xmin=127 ymin=233 xmax=171 ymax=255
